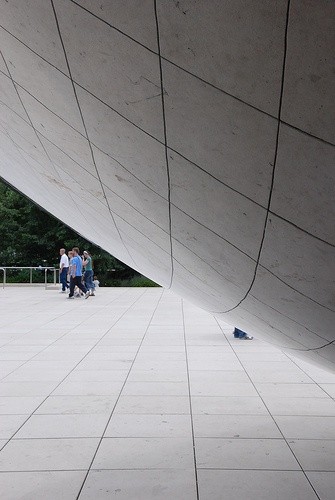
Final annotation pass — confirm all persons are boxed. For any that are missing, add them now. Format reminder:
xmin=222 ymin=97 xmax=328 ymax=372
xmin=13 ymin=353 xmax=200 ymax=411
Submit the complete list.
xmin=68 ymin=250 xmax=85 ymax=297
xmin=80 ymin=249 xmax=95 ymax=296
xmin=232 ymin=327 xmax=253 ymax=341
xmin=67 ymin=248 xmax=90 ymax=300
xmin=59 ymin=248 xmax=70 ymax=292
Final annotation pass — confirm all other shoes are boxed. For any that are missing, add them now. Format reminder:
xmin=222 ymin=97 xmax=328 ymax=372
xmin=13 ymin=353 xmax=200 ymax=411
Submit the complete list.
xmin=85 ymin=291 xmax=90 ymax=299
xmin=60 ymin=290 xmax=65 ymax=293
xmin=89 ymin=293 xmax=95 ymax=296
xmin=66 ymin=296 xmax=74 ymax=299
